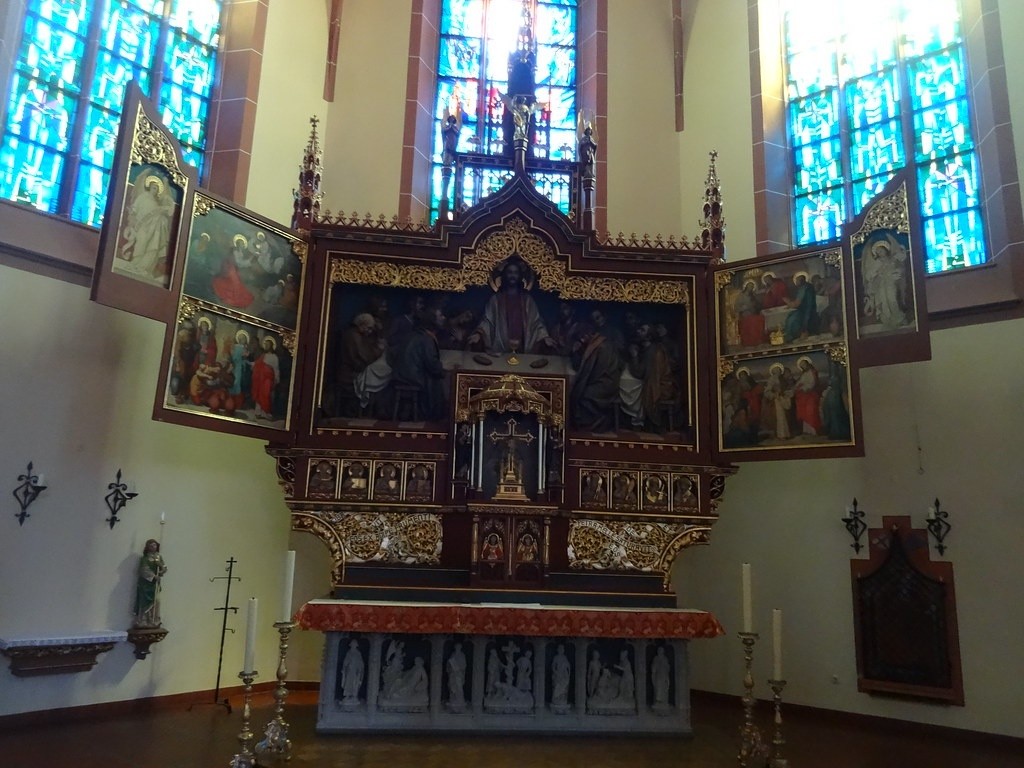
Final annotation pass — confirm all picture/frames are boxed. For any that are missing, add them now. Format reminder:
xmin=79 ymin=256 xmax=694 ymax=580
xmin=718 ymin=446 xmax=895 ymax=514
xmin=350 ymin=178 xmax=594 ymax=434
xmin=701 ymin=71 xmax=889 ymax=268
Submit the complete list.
xmin=716 ymin=240 xmax=844 ymax=358
xmin=315 ymin=250 xmax=698 ymax=452
xmin=718 ymin=347 xmax=850 ymax=453
xmin=181 ymin=189 xmax=307 ymax=337
xmin=162 ymin=290 xmax=289 ymax=431
xmin=110 ymin=148 xmax=188 ymax=293
xmin=853 ymin=225 xmax=920 ymax=340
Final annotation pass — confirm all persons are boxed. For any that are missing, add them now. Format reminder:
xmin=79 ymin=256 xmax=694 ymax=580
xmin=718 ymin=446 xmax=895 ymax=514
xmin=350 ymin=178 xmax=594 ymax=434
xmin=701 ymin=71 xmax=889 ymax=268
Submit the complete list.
xmin=645 ymin=477 xmax=664 ymax=505
xmin=579 ymin=128 xmax=597 ymax=179
xmin=500 ymin=436 xmax=524 ymax=483
xmin=343 ymin=462 xmax=365 ymax=495
xmin=512 ymin=97 xmax=532 ymax=140
xmin=394 ymin=642 xmax=403 ymax=672
xmin=651 ymin=646 xmax=670 ymax=707
xmin=136 ymin=538 xmax=169 ymax=627
xmin=407 ymin=465 xmax=430 ymax=499
xmin=517 ymin=534 xmax=538 ymax=561
xmin=515 ymin=652 xmax=533 ymax=692
xmin=613 ymin=650 xmax=634 ymax=702
xmin=492 ymin=681 xmax=521 ymax=701
xmin=447 ymin=643 xmax=465 ymax=707
xmin=340 ymin=639 xmax=364 ymax=702
xmin=375 ymin=464 xmax=398 ymax=497
xmin=484 ymin=648 xmax=502 ymax=694
xmin=390 ymin=656 xmax=427 ymax=700
xmin=483 ymin=533 xmax=503 ymax=560
xmin=676 ymin=479 xmax=697 ymax=508
xmin=587 ymin=650 xmax=607 ymax=701
xmin=442 ymin=115 xmax=460 ymax=166
xmin=310 ymin=462 xmax=334 ymax=498
xmin=551 ymin=643 xmax=571 ymax=707
xmin=615 ymin=475 xmax=636 ymax=503
xmin=584 ymin=474 xmax=606 ymax=504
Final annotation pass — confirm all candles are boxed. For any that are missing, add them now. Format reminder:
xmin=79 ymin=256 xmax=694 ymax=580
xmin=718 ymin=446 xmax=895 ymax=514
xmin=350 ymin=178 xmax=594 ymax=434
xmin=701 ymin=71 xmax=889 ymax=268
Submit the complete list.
xmin=271 ymin=548 xmax=305 ymax=630
xmin=238 ymin=596 xmax=263 ymax=678
xmin=767 ymin=605 xmax=790 ymax=685
xmin=738 ymin=562 xmax=763 ymax=640
xmin=927 ymin=506 xmax=935 ymax=519
xmin=36 ymin=471 xmax=45 ymax=487
xmin=844 ymin=505 xmax=850 ymax=519
